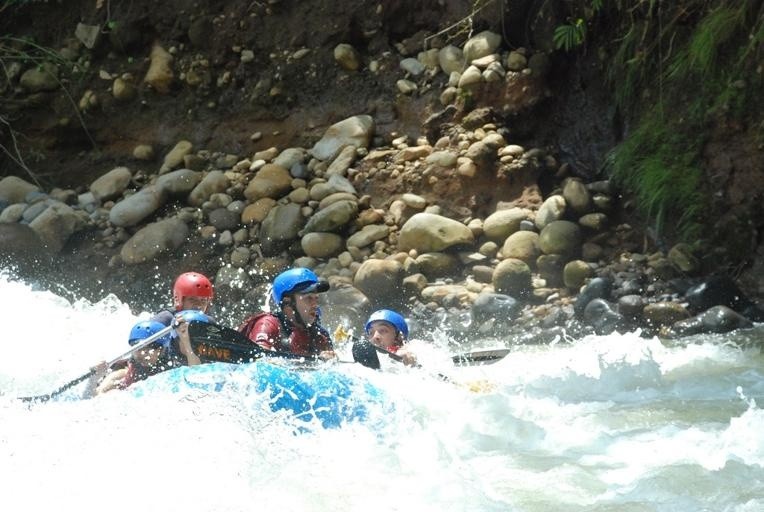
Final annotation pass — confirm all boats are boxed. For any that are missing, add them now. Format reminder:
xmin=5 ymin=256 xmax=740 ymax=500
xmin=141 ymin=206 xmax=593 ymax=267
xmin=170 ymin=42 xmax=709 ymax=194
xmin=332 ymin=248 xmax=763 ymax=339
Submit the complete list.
xmin=44 ymin=360 xmax=429 ymax=451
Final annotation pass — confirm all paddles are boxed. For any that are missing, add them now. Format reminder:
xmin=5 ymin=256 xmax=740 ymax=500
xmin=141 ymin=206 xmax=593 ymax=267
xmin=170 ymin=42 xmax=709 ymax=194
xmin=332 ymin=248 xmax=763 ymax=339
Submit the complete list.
xmin=187 ymin=318 xmax=336 ymax=365
xmin=17 ymin=319 xmax=190 ymax=401
xmin=334 ymin=324 xmax=511 ymax=393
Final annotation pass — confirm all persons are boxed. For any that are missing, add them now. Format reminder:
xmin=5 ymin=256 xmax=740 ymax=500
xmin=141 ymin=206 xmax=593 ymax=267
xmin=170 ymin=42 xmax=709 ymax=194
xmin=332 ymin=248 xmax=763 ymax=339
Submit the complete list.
xmin=352 ymin=309 xmax=418 ymax=371
xmin=150 ymin=270 xmax=214 ymax=328
xmin=80 ymin=314 xmax=202 ymax=400
xmin=234 ymin=267 xmax=339 ymax=366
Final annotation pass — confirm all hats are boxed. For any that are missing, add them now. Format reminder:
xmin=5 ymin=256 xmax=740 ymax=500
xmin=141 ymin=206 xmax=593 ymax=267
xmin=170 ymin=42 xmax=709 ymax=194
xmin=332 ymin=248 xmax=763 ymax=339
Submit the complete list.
xmin=284 ymin=280 xmax=330 ymax=295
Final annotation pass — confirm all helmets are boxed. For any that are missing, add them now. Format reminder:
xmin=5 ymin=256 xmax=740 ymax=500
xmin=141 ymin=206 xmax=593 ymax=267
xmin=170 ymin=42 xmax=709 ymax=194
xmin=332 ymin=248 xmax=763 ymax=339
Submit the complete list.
xmin=273 ymin=267 xmax=330 ymax=304
xmin=174 ymin=271 xmax=213 ymax=310
xmin=366 ymin=310 xmax=408 ymax=343
xmin=128 ymin=320 xmax=170 ymax=349
xmin=170 ymin=310 xmax=210 ymax=339
